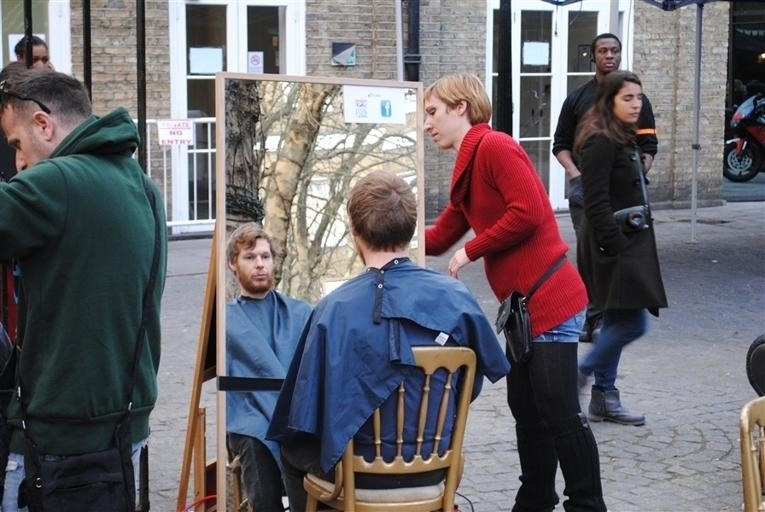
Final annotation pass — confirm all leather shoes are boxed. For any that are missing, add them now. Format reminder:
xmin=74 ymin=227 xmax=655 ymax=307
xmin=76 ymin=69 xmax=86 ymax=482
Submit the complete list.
xmin=579 ymin=322 xmax=591 ymax=342
xmin=591 ymin=322 xmax=602 ymax=344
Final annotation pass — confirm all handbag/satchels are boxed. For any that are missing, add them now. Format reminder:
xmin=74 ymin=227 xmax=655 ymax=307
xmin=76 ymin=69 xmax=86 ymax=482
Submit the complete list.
xmin=16 ymin=444 xmax=138 ymax=511
xmin=495 ymin=289 xmax=533 ymax=365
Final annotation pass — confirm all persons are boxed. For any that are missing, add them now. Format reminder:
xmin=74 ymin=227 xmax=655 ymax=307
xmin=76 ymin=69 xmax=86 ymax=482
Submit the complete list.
xmin=265 ymin=169 xmax=511 ymax=511
xmin=574 ymin=69 xmax=668 ymax=425
xmin=227 ymin=223 xmax=317 ymax=511
xmin=423 ymin=72 xmax=607 ymax=512
xmin=0 ymin=69 xmax=167 ymax=512
xmin=15 ymin=35 xmax=50 ymax=67
xmin=551 ymin=33 xmax=659 ymax=346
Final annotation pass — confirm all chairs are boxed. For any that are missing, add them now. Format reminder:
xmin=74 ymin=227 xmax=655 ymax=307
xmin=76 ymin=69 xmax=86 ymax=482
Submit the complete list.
xmin=739 ymin=396 xmax=765 ymax=512
xmin=302 ymin=346 xmax=478 ymax=512
xmin=229 ymin=454 xmax=253 ymax=511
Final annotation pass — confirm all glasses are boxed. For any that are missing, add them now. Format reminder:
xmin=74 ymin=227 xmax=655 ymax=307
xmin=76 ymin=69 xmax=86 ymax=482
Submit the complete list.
xmin=33 ymin=55 xmax=50 ymax=63
xmin=0 ymin=80 xmax=52 ymax=113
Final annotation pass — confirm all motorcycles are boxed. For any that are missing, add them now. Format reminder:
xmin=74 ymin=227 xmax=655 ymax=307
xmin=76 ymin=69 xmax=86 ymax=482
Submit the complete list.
xmin=724 ymin=93 xmax=765 ymax=183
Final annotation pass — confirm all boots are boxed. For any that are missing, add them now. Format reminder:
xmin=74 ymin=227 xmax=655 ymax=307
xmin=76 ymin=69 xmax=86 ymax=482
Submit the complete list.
xmin=588 ymin=386 xmax=645 ymax=425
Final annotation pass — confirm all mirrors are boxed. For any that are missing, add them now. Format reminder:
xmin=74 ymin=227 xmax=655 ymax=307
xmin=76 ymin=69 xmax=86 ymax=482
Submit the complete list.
xmin=215 ymin=72 xmax=426 ymax=512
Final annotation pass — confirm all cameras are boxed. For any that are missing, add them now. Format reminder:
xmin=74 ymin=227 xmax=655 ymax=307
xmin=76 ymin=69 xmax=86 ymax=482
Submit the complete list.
xmin=614 ymin=205 xmax=648 ymax=233
